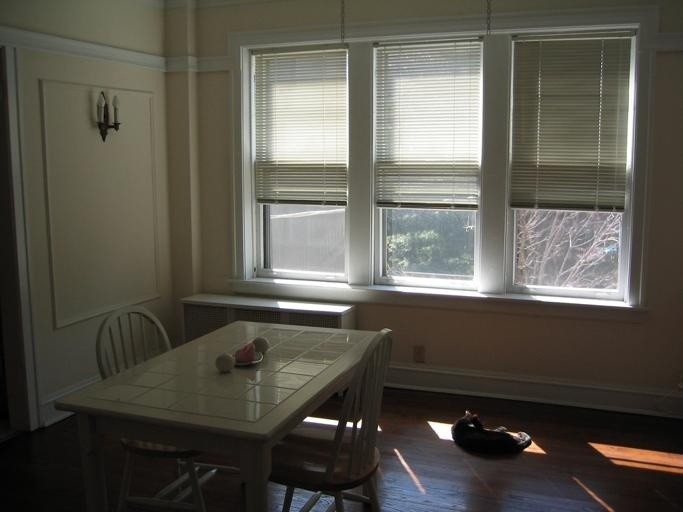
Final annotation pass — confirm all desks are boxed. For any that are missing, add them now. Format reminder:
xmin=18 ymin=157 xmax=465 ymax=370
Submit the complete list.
xmin=51 ymin=317 xmax=390 ymax=512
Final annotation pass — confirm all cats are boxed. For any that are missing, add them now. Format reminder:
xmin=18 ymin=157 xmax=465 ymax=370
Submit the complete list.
xmin=450 ymin=410 xmax=532 ymax=459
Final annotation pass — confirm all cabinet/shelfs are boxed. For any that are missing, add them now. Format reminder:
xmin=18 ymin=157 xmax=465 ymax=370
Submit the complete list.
xmin=179 ymin=292 xmax=357 ymax=346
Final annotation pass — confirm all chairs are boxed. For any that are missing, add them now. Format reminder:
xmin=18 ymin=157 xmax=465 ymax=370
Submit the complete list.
xmin=94 ymin=302 xmax=243 ymax=512
xmin=265 ymin=325 xmax=397 ymax=512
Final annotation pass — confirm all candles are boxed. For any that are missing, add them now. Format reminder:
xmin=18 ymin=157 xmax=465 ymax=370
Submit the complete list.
xmin=96 ymin=93 xmax=104 ymax=121
xmin=111 ymin=95 xmax=119 ymax=122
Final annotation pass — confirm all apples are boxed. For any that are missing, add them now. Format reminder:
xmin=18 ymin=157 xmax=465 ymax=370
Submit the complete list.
xmin=215 ymin=354 xmax=234 ymax=372
xmin=253 ymin=337 xmax=269 ymax=354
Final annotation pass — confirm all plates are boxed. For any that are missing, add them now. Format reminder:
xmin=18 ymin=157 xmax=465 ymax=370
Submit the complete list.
xmin=228 ymin=349 xmax=264 ymax=366
